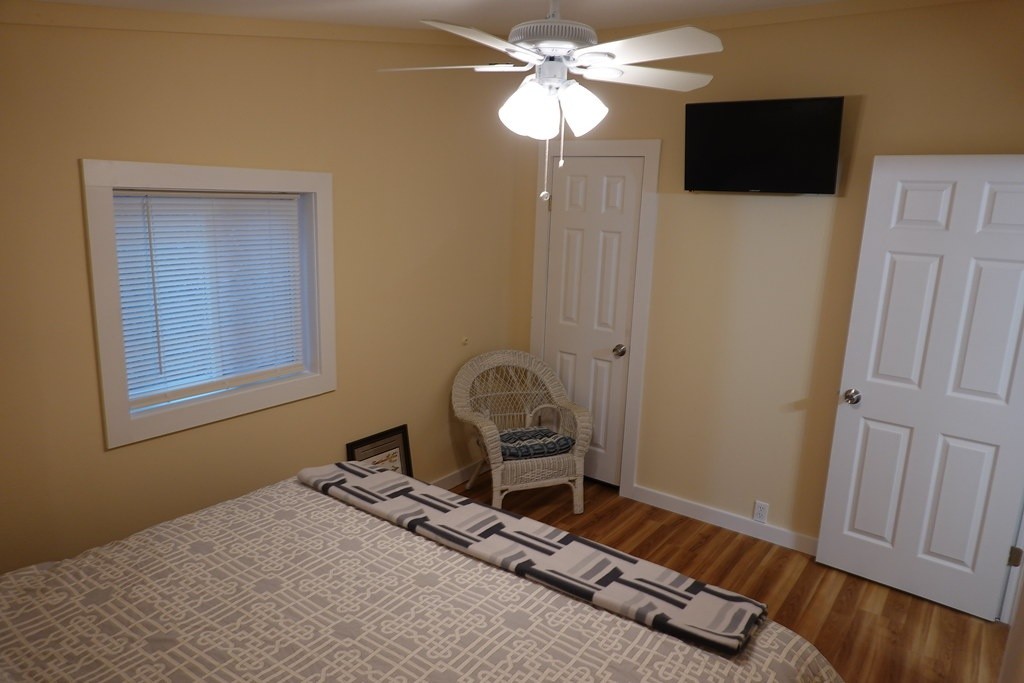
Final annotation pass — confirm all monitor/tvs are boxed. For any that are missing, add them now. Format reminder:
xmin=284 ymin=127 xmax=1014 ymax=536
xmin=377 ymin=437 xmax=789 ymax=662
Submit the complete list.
xmin=685 ymin=96 xmax=844 ymax=194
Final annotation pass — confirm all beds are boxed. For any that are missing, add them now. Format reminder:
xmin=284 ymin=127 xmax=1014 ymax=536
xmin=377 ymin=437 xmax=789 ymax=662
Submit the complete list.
xmin=0 ymin=460 xmax=847 ymax=683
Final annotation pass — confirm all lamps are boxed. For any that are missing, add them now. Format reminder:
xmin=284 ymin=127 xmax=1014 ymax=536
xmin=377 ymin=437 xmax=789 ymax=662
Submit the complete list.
xmin=495 ymin=60 xmax=610 ymax=140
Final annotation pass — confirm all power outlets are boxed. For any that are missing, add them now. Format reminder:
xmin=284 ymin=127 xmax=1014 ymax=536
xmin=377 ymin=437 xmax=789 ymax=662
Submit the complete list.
xmin=753 ymin=500 xmax=769 ymax=524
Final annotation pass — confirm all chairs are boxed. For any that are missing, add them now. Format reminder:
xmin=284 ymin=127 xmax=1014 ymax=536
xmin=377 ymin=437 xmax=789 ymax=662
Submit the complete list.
xmin=449 ymin=348 xmax=594 ymax=513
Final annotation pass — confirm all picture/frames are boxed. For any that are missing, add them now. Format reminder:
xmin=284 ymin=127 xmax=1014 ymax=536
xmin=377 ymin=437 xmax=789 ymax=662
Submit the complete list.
xmin=345 ymin=424 xmax=415 ymax=477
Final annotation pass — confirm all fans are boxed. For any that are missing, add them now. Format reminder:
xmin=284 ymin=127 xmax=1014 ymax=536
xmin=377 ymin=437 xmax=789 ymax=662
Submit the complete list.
xmin=377 ymin=0 xmax=723 ymax=94
